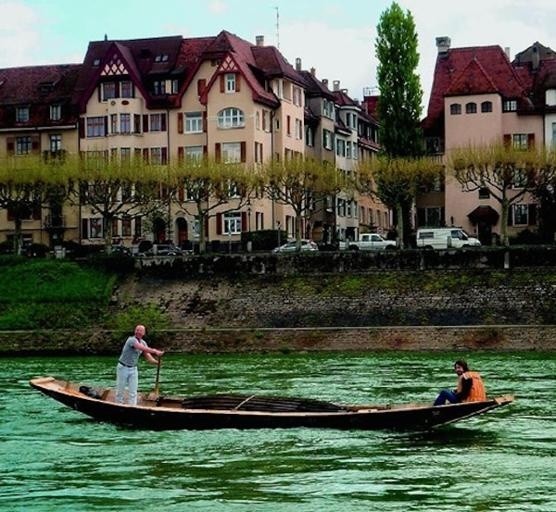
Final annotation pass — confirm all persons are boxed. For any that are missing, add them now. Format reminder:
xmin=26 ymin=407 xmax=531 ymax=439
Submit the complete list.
xmin=114 ymin=324 xmax=164 ymax=405
xmin=433 ymin=360 xmax=486 ymax=407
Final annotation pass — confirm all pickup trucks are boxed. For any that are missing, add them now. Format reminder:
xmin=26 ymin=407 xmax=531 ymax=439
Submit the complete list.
xmin=338 ymin=232 xmax=399 ymax=252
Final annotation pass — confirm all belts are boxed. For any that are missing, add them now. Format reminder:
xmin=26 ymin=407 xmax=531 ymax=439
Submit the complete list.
xmin=118 ymin=361 xmax=132 ymax=368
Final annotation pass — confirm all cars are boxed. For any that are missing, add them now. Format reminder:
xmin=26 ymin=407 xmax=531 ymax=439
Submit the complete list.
xmin=271 ymin=238 xmax=320 ymax=253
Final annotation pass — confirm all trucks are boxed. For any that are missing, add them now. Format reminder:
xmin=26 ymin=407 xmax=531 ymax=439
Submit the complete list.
xmin=415 ymin=227 xmax=483 ymax=251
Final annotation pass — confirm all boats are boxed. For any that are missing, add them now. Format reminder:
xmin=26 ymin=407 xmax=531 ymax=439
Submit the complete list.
xmin=28 ymin=375 xmax=515 ymax=435
